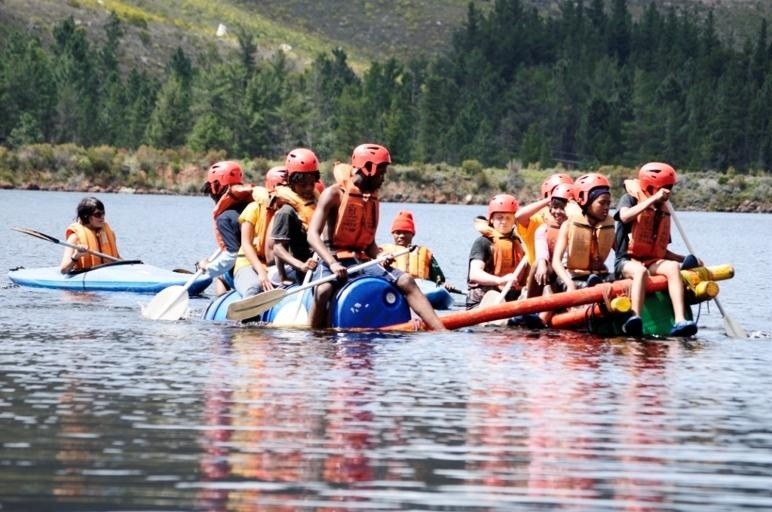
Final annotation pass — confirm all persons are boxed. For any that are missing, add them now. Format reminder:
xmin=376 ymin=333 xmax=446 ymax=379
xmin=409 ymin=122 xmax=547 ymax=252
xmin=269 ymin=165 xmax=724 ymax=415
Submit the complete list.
xmin=233 ymin=166 xmax=287 ymax=324
xmin=614 ymin=162 xmax=704 ymax=339
xmin=378 ymin=211 xmax=454 ymax=291
xmin=551 ymin=174 xmax=617 ymax=330
xmin=198 ymin=160 xmax=255 ymax=298
xmin=522 ymin=183 xmax=576 ymax=329
xmin=306 ymin=144 xmax=451 ymax=333
xmin=466 ymin=193 xmax=531 ymax=311
xmin=269 ymin=148 xmax=320 ymax=287
xmin=514 ymin=173 xmax=574 ymax=300
xmin=60 ymin=197 xmax=124 ymax=275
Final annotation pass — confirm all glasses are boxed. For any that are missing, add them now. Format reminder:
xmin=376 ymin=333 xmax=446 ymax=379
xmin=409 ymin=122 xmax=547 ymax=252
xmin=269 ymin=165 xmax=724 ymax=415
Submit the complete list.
xmin=91 ymin=211 xmax=104 ymax=218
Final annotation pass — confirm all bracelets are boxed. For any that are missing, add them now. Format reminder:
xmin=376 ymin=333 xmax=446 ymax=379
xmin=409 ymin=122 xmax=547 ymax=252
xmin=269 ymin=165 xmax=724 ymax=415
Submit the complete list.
xmin=72 ymin=258 xmax=78 ymax=263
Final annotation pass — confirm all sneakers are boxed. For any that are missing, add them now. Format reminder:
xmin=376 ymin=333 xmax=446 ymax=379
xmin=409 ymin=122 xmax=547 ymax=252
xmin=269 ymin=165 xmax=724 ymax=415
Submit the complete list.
xmin=670 ymin=320 xmax=697 ymax=338
xmin=588 ymin=274 xmax=602 ymax=286
xmin=508 ymin=313 xmax=541 ymax=328
xmin=682 ymin=255 xmax=699 ymax=269
xmin=625 ymin=317 xmax=644 ymax=339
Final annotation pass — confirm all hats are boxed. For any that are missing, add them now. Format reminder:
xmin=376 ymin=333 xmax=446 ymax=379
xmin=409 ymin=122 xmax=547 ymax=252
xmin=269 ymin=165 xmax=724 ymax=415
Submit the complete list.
xmin=391 ymin=212 xmax=415 ymax=234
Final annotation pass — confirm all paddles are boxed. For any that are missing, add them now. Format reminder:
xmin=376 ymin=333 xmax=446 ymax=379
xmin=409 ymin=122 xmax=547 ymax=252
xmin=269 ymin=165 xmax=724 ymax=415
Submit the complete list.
xmin=145 ymin=245 xmax=222 ymax=322
xmin=664 ymin=199 xmax=750 ymax=339
xmin=10 ymin=226 xmax=124 ymax=261
xmin=475 ymin=255 xmax=530 ymax=326
xmin=226 ymin=244 xmax=419 ymax=322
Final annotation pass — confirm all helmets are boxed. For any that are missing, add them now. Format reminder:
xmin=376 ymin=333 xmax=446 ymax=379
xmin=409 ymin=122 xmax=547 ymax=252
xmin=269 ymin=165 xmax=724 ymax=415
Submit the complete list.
xmin=489 ymin=162 xmax=677 ymax=224
xmin=200 ymin=144 xmax=391 ymax=203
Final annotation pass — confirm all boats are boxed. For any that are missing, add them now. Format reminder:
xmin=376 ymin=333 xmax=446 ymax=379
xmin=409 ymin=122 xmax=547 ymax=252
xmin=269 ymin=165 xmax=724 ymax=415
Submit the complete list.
xmin=7 ymin=259 xmax=212 ymax=296
xmin=200 ymin=276 xmax=411 ymax=330
xmin=384 ymin=261 xmax=736 ymax=344
xmin=414 ymin=279 xmax=454 ymax=310
